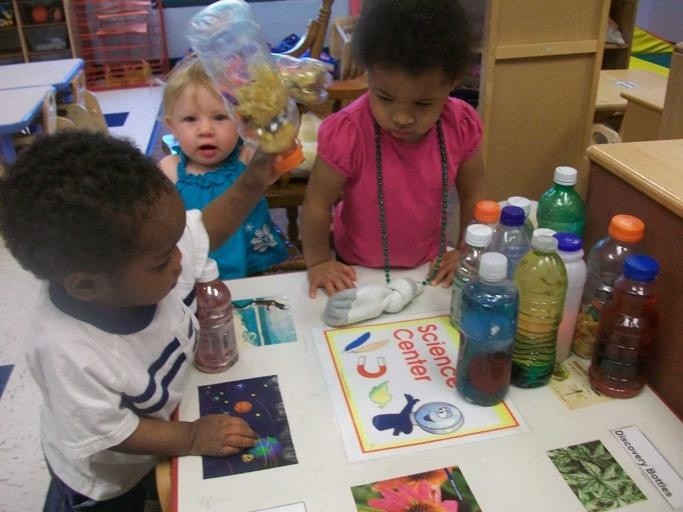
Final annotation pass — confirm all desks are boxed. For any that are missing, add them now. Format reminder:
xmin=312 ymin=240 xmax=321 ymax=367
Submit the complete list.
xmin=154 ymin=258 xmax=683 ymax=512
xmin=0 ymin=85 xmax=109 ymax=167
xmin=0 ymin=57 xmax=86 ymax=103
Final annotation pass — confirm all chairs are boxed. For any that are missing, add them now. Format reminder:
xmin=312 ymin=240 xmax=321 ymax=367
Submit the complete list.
xmin=162 ymin=0 xmax=369 ymax=253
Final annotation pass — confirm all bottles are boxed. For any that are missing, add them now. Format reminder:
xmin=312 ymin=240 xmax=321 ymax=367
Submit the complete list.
xmin=263 ymin=50 xmax=333 ymax=108
xmin=574 ymin=212 xmax=643 ymax=359
xmin=193 ymin=258 xmax=241 ymax=375
xmin=589 ymin=255 xmax=660 ymax=402
xmin=186 ymin=0 xmax=308 ymax=174
xmin=453 ymin=163 xmax=586 ymax=406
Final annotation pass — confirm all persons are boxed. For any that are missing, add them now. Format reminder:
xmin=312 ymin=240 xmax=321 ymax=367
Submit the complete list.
xmin=0 ymin=125 xmax=291 ymax=510
xmin=301 ymin=1 xmax=489 ymax=301
xmin=146 ymin=49 xmax=293 ymax=288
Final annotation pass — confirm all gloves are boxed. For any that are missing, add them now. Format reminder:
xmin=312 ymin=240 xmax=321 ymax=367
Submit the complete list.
xmin=322 ymin=276 xmax=419 ymax=328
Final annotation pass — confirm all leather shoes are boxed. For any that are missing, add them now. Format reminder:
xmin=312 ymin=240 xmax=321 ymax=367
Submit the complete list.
xmin=35 ymin=38 xmax=66 ymax=51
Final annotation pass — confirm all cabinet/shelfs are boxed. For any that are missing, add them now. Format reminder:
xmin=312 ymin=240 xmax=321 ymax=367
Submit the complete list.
xmin=0 ymin=0 xmax=79 ymax=65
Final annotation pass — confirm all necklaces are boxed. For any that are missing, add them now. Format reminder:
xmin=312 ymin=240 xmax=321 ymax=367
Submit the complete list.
xmin=365 ymin=113 xmax=450 ymax=289
